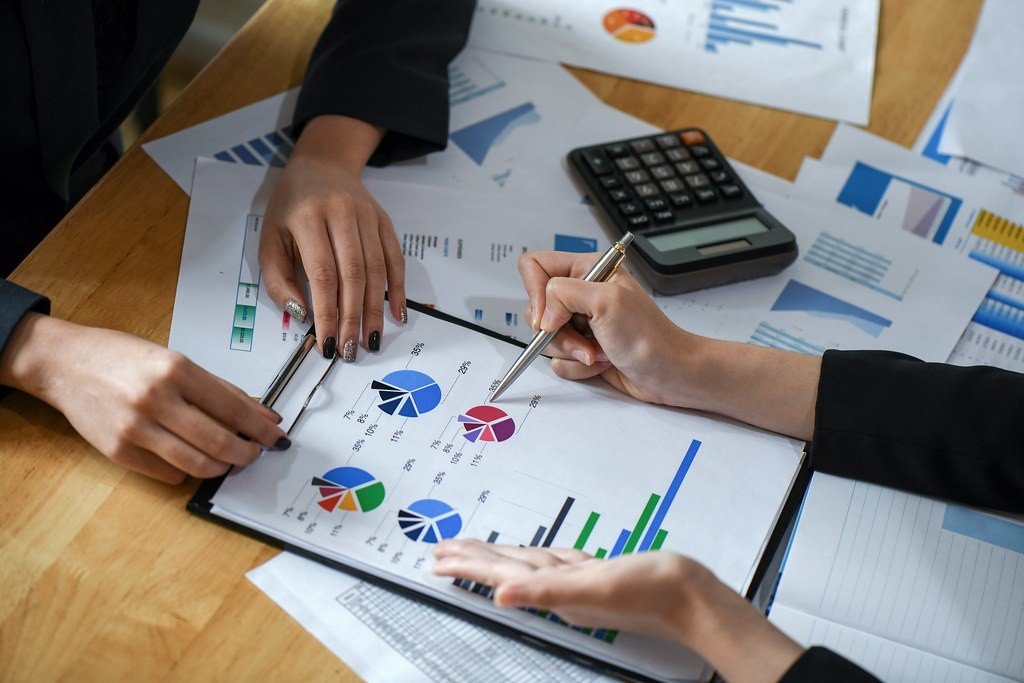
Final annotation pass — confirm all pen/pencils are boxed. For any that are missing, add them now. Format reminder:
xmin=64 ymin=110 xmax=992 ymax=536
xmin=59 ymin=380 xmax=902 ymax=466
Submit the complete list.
xmin=489 ymin=231 xmax=636 ymax=404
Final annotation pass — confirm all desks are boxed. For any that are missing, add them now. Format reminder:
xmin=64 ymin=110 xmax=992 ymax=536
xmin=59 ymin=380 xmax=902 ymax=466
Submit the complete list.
xmin=2 ymin=0 xmax=1024 ymax=681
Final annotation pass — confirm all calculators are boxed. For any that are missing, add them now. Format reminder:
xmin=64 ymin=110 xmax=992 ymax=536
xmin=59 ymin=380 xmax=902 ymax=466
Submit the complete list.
xmin=566 ymin=127 xmax=799 ymax=296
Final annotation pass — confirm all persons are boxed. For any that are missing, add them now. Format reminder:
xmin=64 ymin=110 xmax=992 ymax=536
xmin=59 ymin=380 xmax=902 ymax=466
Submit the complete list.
xmin=435 ymin=251 xmax=1024 ymax=683
xmin=0 ymin=0 xmax=479 ymax=488
